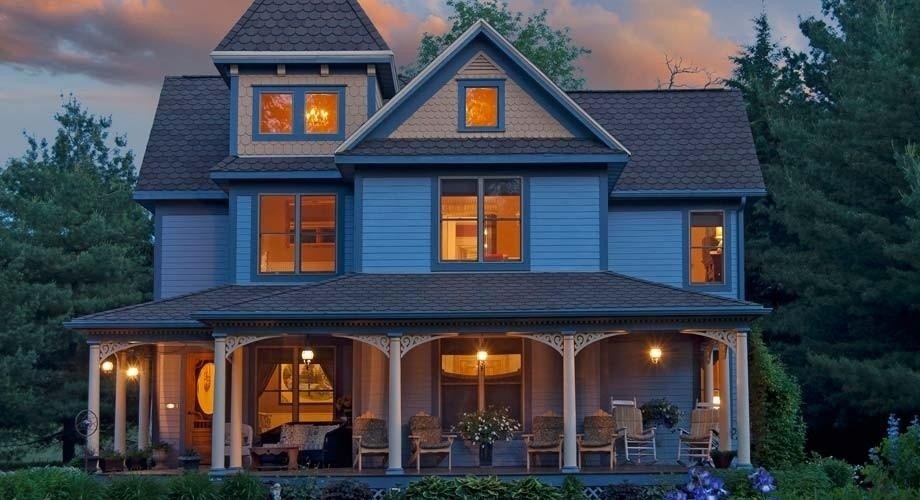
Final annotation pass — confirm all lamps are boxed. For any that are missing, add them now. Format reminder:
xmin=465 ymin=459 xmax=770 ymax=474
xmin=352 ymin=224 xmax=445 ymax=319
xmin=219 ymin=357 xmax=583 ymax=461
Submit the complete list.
xmin=476 ymin=350 xmax=488 ymax=368
xmin=302 ymin=350 xmax=314 ymax=370
xmin=650 ymin=349 xmax=662 ymax=365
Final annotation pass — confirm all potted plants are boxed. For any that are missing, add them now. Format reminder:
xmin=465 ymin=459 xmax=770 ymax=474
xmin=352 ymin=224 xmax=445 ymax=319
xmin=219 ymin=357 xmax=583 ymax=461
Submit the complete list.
xmin=452 ymin=404 xmax=520 ymax=467
xmin=73 ymin=439 xmax=202 ymax=473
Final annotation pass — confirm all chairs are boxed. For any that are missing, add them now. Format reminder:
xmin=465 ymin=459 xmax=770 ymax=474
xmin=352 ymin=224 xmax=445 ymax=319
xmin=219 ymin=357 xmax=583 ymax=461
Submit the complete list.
xmin=521 ymin=397 xmax=723 ymax=471
xmin=217 ymin=411 xmax=457 ymax=473
